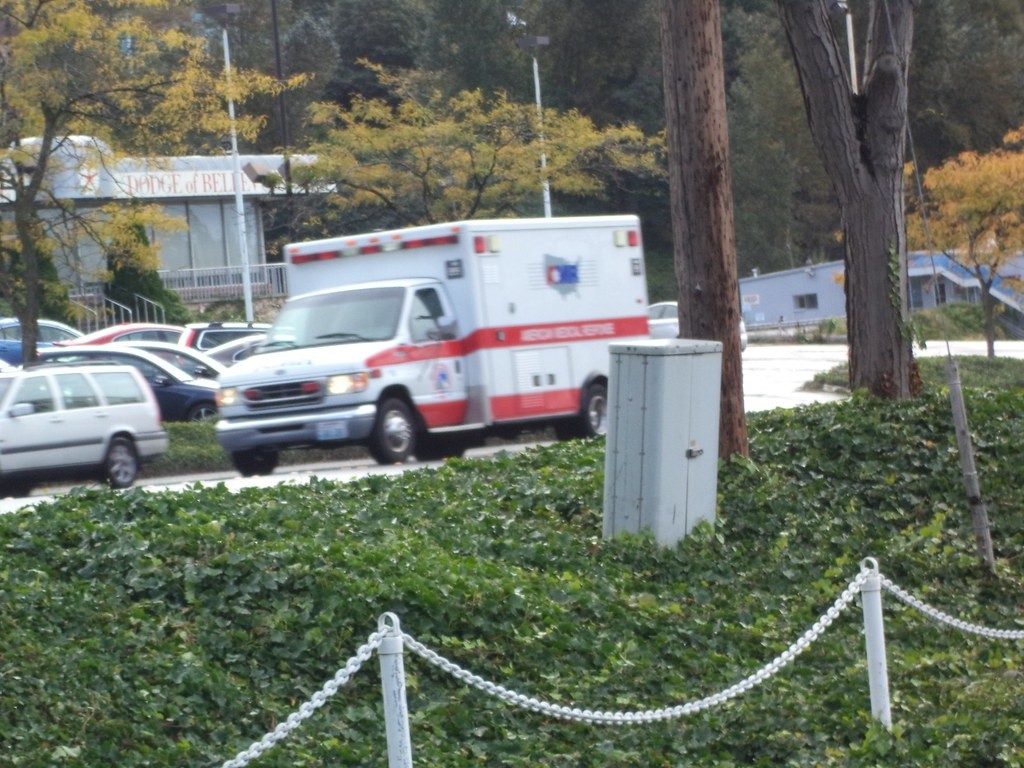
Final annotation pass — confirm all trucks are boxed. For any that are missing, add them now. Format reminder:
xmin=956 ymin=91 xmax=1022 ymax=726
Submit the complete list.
xmin=210 ymin=214 xmax=654 ymax=476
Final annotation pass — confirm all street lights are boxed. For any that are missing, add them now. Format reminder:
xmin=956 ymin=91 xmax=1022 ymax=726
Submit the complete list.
xmin=506 ymin=6 xmax=552 ymax=219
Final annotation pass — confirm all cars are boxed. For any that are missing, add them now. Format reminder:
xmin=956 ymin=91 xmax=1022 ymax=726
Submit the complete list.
xmin=0 ymin=316 xmax=275 ymax=423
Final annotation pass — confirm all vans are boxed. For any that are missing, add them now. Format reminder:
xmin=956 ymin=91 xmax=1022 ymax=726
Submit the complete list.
xmin=0 ymin=361 xmax=169 ymax=488
